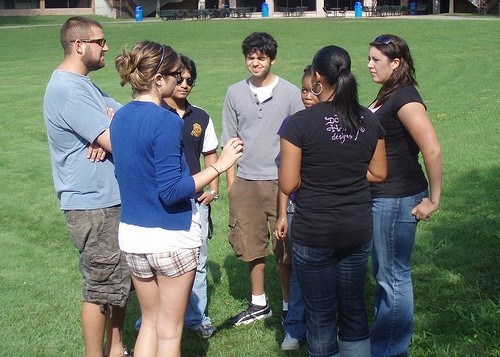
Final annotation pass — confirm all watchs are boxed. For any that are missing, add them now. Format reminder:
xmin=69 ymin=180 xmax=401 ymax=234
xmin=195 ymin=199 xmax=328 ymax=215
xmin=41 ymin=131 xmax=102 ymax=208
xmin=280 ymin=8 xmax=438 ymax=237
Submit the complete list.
xmin=209 ymin=189 xmax=219 ymax=201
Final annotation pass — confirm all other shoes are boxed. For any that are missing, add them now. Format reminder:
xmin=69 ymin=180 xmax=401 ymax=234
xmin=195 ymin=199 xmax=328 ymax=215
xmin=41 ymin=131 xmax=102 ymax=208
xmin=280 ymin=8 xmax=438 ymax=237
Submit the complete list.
xmin=188 ymin=322 xmax=217 ymax=339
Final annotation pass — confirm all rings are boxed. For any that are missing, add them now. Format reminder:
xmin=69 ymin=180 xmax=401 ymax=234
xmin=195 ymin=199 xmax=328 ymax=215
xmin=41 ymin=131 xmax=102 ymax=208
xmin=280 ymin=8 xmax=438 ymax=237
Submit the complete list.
xmin=233 ymin=144 xmax=236 ymax=148
xmin=236 ymin=148 xmax=239 ymax=153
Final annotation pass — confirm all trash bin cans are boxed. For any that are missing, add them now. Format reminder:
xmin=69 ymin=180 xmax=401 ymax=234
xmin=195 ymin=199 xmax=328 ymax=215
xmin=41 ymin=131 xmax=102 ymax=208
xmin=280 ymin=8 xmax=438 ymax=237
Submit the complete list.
xmin=410 ymin=2 xmax=415 ymax=15
xmin=354 ymin=2 xmax=362 ymax=17
xmin=135 ymin=6 xmax=142 ymax=20
xmin=225 ymin=5 xmax=229 ymax=16
xmin=262 ymin=3 xmax=268 ymax=17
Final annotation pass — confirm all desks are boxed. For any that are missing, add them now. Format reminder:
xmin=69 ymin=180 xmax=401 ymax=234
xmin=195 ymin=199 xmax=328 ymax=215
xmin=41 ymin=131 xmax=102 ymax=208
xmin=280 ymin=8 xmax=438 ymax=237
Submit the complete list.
xmin=330 ymin=8 xmax=341 ymax=17
xmin=289 ymin=8 xmax=295 ymax=16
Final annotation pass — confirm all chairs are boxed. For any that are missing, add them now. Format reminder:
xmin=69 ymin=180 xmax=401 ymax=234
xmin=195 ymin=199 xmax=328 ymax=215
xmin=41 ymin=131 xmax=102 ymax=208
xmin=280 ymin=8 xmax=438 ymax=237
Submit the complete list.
xmin=160 ymin=7 xmax=257 ymax=21
xmin=363 ymin=6 xmax=408 ymax=17
xmin=323 ymin=7 xmax=332 ymax=18
xmin=339 ymin=7 xmax=348 ymax=17
xmin=277 ymin=6 xmax=307 ymax=16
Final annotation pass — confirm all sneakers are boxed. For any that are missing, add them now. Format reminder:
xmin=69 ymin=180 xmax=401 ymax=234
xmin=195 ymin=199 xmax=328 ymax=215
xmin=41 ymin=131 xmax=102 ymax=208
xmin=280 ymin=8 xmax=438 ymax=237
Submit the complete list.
xmin=281 ymin=310 xmax=287 ymax=325
xmin=227 ymin=303 xmax=273 ymax=327
xmin=281 ymin=333 xmax=299 ymax=351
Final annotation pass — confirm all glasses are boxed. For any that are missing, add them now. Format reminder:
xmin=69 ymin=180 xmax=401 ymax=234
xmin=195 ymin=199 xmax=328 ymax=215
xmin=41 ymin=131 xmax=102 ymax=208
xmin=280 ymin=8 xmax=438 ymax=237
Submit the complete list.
xmin=161 ymin=69 xmax=182 ymax=79
xmin=69 ymin=38 xmax=106 ymax=48
xmin=373 ymin=36 xmax=391 ymax=45
xmin=176 ymin=77 xmax=193 ymax=86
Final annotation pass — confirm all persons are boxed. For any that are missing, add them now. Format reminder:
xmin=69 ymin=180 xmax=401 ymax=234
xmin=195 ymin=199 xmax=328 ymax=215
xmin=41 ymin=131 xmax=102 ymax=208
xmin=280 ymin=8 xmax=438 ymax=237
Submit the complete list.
xmin=135 ymin=52 xmax=220 ymax=339
xmin=366 ymin=34 xmax=443 ymax=357
xmin=211 ymin=31 xmax=307 ymax=326
xmin=274 ymin=64 xmax=320 ymax=350
xmin=108 ymin=39 xmax=244 ymax=357
xmin=278 ymin=45 xmax=388 ymax=357
xmin=43 ymin=15 xmax=135 ymax=357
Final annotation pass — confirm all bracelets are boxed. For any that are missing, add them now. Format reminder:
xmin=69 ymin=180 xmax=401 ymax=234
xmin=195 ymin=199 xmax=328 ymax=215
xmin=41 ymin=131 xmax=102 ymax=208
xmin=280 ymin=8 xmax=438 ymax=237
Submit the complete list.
xmin=276 ymin=217 xmax=287 ymax=222
xmin=209 ymin=165 xmax=220 ymax=176
